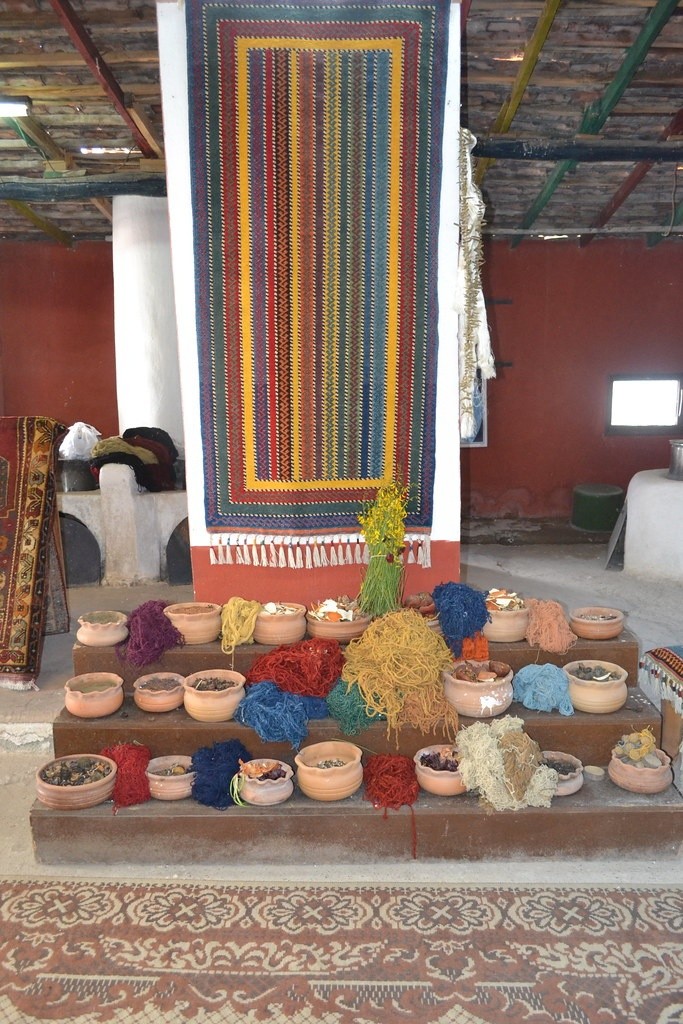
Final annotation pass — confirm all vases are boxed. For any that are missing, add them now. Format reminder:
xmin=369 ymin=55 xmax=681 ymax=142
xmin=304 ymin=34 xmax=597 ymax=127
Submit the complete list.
xmin=36 ymin=587 xmax=678 ymax=808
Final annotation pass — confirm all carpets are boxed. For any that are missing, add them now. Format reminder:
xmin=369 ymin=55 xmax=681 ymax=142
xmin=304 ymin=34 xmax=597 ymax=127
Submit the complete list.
xmin=0 ymin=877 xmax=683 ymax=1024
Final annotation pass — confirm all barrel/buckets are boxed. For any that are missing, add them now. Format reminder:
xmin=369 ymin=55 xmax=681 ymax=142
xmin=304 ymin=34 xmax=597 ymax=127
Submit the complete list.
xmin=666 ymin=439 xmax=683 ymax=481
xmin=569 ymin=483 xmax=625 ymax=534
xmin=56 ymin=457 xmax=96 ymax=492
